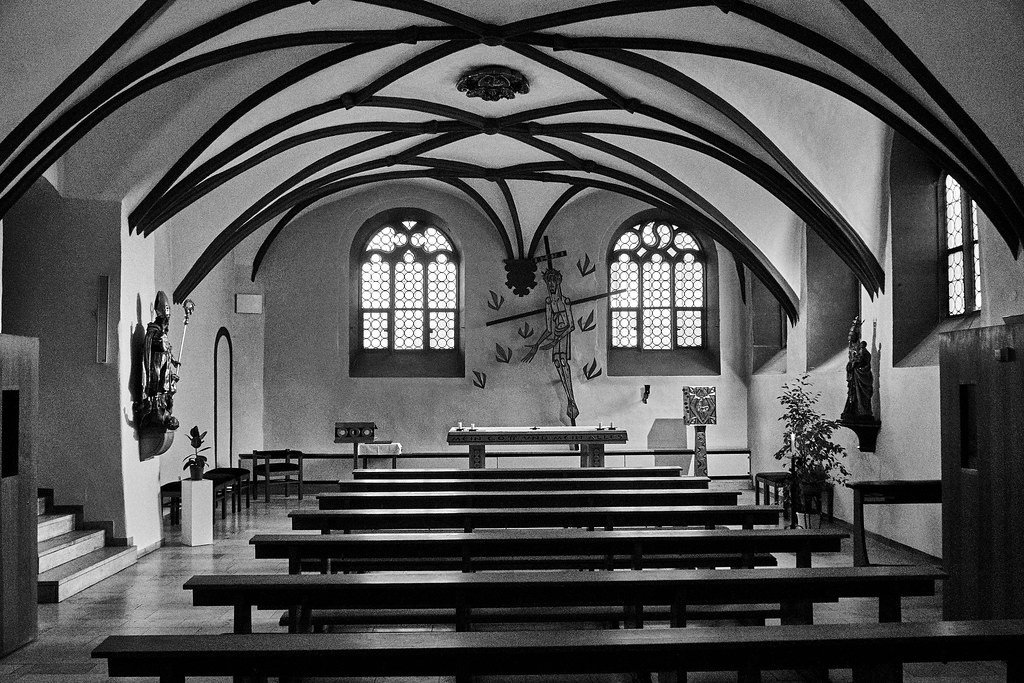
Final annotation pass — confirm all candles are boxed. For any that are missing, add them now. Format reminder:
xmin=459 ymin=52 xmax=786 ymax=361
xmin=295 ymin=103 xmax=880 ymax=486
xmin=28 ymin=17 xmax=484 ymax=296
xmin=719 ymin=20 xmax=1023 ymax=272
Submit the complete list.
xmin=611 ymin=422 xmax=615 ymax=428
xmin=458 ymin=422 xmax=463 ymax=429
xmin=599 ymin=422 xmax=602 ymax=428
xmin=471 ymin=423 xmax=476 ymax=430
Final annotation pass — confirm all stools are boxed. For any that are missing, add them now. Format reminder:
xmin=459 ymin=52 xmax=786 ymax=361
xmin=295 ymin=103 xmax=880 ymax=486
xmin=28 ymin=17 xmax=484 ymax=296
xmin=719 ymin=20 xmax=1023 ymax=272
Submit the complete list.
xmin=253 ymin=448 xmax=307 ymax=502
xmin=204 ymin=474 xmax=234 ymax=519
xmin=160 ymin=479 xmax=183 ymax=526
xmin=797 ymin=481 xmax=836 ymax=520
xmin=215 ymin=464 xmax=251 ymax=512
xmin=765 ymin=474 xmax=789 ymax=504
xmin=753 ymin=470 xmax=789 ymax=508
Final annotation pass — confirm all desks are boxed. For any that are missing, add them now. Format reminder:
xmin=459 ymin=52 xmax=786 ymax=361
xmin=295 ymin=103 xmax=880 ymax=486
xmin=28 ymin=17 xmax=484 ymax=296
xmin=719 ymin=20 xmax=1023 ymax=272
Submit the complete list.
xmin=358 ymin=442 xmax=402 ymax=468
xmin=446 ymin=426 xmax=630 ymax=467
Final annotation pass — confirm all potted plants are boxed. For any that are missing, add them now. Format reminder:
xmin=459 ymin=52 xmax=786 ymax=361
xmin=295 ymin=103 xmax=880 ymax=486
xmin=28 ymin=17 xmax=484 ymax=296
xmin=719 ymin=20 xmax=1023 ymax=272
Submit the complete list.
xmin=179 ymin=424 xmax=211 ymax=480
xmin=772 ymin=367 xmax=853 ymax=515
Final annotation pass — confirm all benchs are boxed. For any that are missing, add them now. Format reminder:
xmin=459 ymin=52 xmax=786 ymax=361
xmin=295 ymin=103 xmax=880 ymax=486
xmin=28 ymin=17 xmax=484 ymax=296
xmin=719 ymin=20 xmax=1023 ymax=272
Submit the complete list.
xmin=83 ymin=464 xmax=1024 ymax=683
